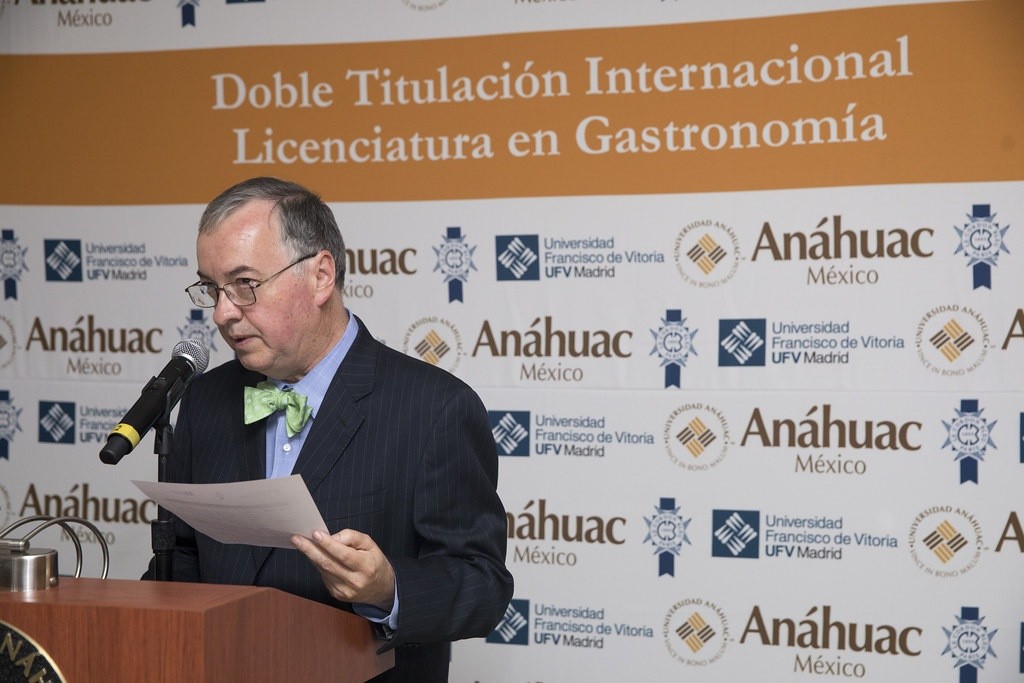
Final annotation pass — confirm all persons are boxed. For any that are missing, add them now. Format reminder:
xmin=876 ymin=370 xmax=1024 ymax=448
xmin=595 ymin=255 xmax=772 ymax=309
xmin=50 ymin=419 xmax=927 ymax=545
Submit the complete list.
xmin=138 ymin=176 xmax=514 ymax=683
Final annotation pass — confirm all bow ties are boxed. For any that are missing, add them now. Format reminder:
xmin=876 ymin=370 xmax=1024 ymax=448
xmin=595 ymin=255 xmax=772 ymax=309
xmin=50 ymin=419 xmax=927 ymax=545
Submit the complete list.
xmin=244 ymin=381 xmax=312 ymax=439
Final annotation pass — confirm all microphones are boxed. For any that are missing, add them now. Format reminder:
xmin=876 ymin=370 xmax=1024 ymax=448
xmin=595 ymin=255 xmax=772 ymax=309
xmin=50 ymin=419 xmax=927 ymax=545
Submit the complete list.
xmin=100 ymin=339 xmax=210 ymax=465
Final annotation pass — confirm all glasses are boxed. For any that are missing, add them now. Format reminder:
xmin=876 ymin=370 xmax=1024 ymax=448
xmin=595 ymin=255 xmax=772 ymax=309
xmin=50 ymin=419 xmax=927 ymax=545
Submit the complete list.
xmin=184 ymin=251 xmax=318 ymax=309
